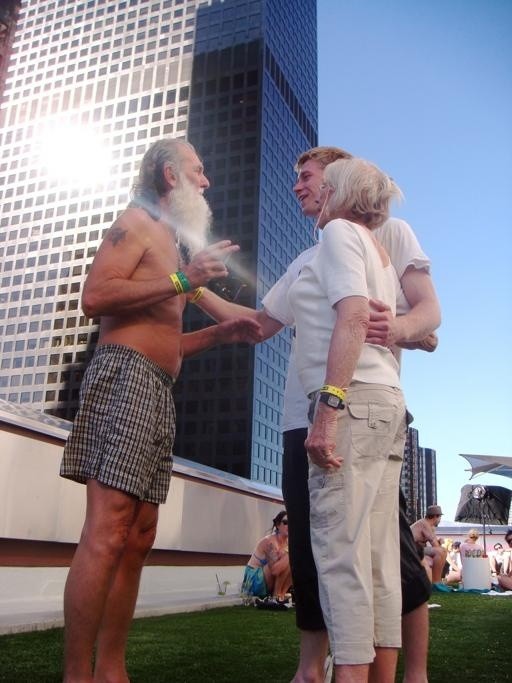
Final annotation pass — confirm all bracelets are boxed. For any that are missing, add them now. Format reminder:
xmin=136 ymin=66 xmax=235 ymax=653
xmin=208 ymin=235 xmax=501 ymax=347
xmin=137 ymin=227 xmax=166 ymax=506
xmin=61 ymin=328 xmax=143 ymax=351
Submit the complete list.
xmin=189 ymin=287 xmax=204 ymax=304
xmin=176 ymin=271 xmax=190 ymax=293
xmin=169 ymin=273 xmax=184 ymax=294
xmin=317 ymin=384 xmax=346 ymax=400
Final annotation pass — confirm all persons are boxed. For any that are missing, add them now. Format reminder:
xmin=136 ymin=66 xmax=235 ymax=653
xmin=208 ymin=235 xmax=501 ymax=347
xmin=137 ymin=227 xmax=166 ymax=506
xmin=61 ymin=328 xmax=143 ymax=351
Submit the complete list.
xmin=184 ymin=144 xmax=443 ymax=681
xmin=59 ymin=135 xmax=263 ymax=680
xmin=409 ymin=506 xmax=511 ymax=593
xmin=287 ymin=156 xmax=404 ymax=683
xmin=242 ymin=512 xmax=293 ymax=607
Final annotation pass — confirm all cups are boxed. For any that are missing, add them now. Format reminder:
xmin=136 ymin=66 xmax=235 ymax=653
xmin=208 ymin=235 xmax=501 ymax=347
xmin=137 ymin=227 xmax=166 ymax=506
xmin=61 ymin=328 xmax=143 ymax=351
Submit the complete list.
xmin=216 ymin=582 xmax=228 ymax=595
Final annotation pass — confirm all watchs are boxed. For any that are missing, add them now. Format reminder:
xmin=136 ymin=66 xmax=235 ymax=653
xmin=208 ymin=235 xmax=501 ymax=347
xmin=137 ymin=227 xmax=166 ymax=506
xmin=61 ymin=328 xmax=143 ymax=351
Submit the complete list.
xmin=319 ymin=392 xmax=345 ymax=411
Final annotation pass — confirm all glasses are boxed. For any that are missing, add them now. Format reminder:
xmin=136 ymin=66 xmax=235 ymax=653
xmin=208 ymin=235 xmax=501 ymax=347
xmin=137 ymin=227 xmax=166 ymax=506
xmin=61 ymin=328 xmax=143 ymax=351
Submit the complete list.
xmin=281 ymin=520 xmax=287 ymax=525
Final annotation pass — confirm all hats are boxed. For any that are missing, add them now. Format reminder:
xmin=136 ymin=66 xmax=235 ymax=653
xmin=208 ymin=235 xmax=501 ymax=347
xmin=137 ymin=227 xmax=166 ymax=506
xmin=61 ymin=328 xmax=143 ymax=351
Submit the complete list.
xmin=427 ymin=506 xmax=443 ymax=515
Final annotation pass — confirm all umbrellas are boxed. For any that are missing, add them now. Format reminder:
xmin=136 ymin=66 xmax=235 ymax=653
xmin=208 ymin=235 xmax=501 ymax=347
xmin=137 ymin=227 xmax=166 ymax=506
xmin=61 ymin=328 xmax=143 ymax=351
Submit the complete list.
xmin=459 ymin=452 xmax=512 ymax=480
